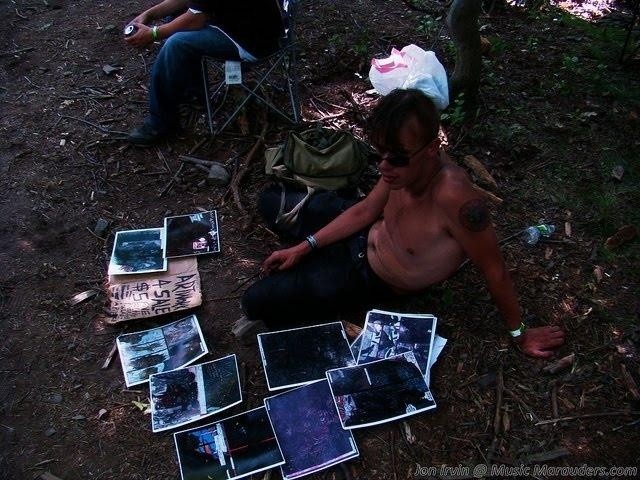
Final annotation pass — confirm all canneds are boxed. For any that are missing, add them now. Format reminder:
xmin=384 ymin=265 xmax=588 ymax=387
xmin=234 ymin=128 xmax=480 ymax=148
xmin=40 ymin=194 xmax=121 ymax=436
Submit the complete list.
xmin=123 ymin=25 xmax=136 ymax=38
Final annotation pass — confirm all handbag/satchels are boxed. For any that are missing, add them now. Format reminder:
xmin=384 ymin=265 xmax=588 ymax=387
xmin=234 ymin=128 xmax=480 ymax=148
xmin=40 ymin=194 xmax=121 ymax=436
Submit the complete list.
xmin=264 ymin=128 xmax=363 ymax=191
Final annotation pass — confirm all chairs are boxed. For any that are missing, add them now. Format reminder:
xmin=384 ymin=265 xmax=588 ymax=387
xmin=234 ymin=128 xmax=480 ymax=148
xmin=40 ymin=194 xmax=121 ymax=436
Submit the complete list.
xmin=201 ymin=0 xmax=301 ymax=143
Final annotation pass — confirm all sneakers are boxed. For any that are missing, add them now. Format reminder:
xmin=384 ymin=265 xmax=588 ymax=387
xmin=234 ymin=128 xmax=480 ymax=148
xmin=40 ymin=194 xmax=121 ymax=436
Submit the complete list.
xmin=127 ymin=119 xmax=182 ymax=145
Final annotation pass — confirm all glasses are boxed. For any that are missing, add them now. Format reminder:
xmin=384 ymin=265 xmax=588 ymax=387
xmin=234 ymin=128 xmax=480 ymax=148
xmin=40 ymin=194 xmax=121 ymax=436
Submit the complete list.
xmin=378 ymin=141 xmax=430 ymax=167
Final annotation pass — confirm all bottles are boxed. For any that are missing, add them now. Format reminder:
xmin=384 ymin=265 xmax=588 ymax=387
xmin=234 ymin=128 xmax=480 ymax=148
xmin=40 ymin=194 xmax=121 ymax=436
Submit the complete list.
xmin=523 ymin=222 xmax=556 ymax=246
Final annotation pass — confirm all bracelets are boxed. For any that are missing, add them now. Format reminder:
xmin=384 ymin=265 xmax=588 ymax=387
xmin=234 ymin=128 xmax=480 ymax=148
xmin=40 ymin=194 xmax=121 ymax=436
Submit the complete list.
xmin=509 ymin=322 xmax=527 ymax=338
xmin=306 ymin=234 xmax=319 ymax=249
xmin=151 ymin=26 xmax=159 ymax=42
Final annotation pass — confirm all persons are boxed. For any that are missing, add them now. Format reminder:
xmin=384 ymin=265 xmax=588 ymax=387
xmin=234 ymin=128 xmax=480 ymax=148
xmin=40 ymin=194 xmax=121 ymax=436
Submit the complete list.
xmin=122 ymin=0 xmax=285 ymax=147
xmin=242 ymin=87 xmax=565 ymax=360
